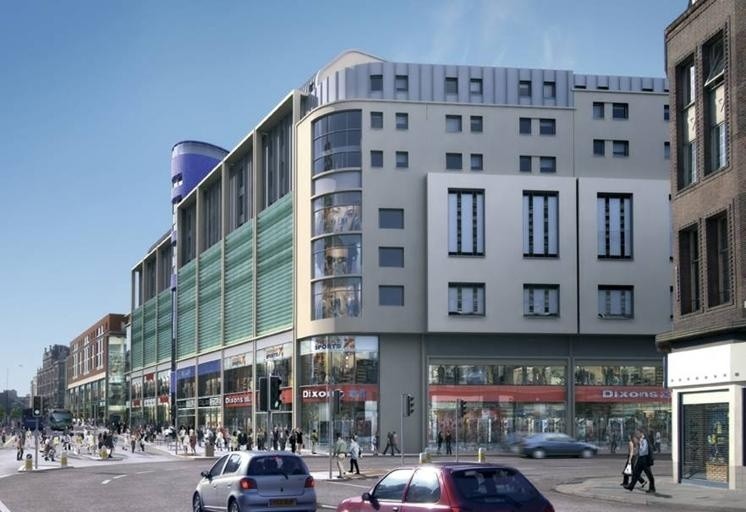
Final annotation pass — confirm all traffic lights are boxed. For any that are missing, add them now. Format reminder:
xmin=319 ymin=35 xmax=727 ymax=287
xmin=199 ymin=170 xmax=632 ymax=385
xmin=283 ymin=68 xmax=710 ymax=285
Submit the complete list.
xmin=410 ymin=396 xmax=415 ymax=412
xmin=34 ymin=396 xmax=40 ymax=415
xmin=340 ymin=390 xmax=344 ymax=411
xmin=463 ymin=400 xmax=468 ymax=416
xmin=278 ymin=378 xmax=283 ymax=407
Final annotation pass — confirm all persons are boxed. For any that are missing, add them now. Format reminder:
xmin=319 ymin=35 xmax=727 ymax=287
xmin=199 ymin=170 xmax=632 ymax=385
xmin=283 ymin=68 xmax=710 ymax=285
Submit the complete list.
xmin=316 ymin=236 xmax=359 ymax=275
xmin=439 ymin=364 xmax=629 ymax=384
xmin=318 ymin=203 xmax=362 ymax=234
xmin=611 ymin=429 xmax=661 ymax=493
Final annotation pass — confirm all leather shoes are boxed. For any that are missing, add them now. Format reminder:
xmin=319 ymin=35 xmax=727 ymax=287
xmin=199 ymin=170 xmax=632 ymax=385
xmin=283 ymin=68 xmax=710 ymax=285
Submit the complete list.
xmin=620 ymin=481 xmax=656 ymax=493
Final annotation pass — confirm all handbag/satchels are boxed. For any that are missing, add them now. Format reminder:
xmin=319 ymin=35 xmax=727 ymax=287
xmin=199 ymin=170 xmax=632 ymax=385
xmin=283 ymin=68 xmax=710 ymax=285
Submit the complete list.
xmin=624 ymin=463 xmax=632 ymax=475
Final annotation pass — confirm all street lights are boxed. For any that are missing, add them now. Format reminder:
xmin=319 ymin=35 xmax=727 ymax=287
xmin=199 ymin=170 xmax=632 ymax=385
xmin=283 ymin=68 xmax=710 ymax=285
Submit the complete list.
xmin=5 ymin=364 xmax=24 ymax=422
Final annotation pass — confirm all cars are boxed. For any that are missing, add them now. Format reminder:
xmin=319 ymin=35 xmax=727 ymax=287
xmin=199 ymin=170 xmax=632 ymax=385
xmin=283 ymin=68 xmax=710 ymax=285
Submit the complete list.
xmin=335 ymin=461 xmax=555 ymax=511
xmin=510 ymin=431 xmax=598 ymax=459
xmin=187 ymin=448 xmax=318 ymax=512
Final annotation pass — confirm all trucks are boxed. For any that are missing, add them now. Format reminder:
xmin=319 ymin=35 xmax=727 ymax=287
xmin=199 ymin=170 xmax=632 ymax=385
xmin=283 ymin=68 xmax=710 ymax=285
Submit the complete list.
xmin=48 ymin=407 xmax=72 ymax=431
xmin=22 ymin=406 xmax=43 ymax=431
